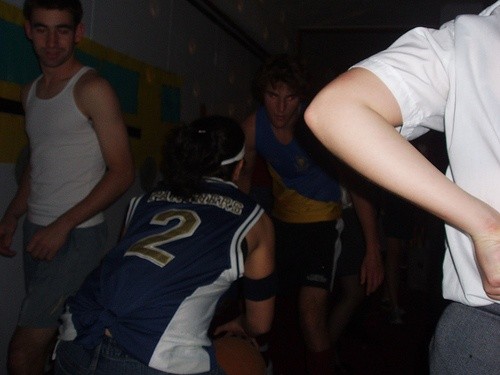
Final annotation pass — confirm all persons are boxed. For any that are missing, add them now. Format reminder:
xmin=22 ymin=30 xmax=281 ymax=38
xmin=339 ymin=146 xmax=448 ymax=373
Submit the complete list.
xmin=0 ymin=0 xmax=136 ymax=375
xmin=50 ymin=0 xmax=500 ymax=375
